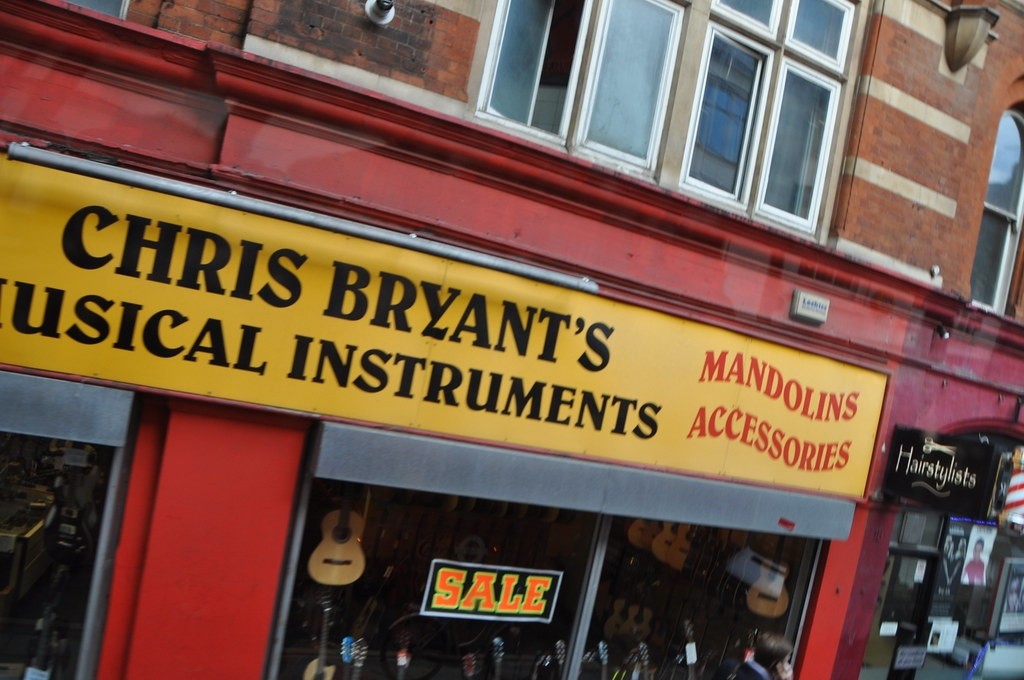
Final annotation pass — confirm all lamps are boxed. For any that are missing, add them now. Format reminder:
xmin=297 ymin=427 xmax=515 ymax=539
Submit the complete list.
xmin=925 ymin=0 xmax=1000 ymax=74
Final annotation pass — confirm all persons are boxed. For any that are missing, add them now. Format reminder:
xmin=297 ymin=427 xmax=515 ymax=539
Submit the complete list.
xmin=937 ymin=534 xmax=967 ymax=595
xmin=711 ymin=630 xmax=794 ymax=680
xmin=962 ymin=538 xmax=984 ymax=585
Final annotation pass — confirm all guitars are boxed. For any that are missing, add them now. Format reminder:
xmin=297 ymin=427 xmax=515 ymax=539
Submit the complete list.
xmin=293 ymin=469 xmax=801 ymax=680
